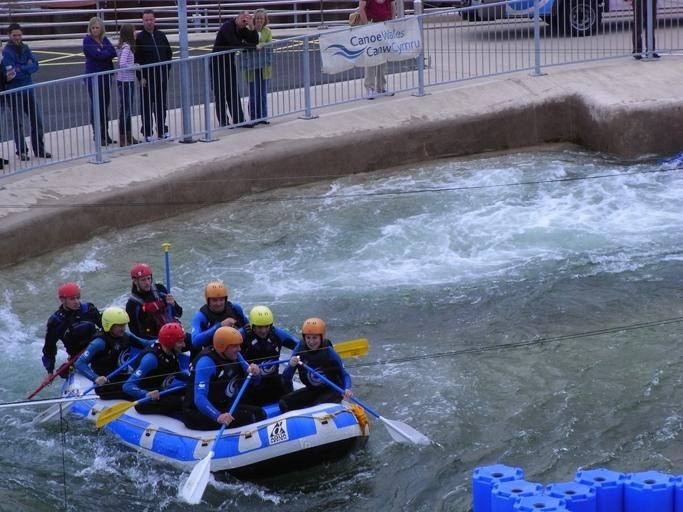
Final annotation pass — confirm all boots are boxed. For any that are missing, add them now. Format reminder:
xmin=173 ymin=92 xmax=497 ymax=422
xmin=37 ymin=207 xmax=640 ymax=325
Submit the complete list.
xmin=120 ymin=134 xmax=126 ymax=147
xmin=128 ymin=130 xmax=142 ymax=144
xmin=90 ymin=117 xmax=117 ymax=146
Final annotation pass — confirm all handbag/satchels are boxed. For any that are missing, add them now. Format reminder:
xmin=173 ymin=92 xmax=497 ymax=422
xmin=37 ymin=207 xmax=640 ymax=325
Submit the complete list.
xmin=349 ymin=12 xmax=361 ymax=26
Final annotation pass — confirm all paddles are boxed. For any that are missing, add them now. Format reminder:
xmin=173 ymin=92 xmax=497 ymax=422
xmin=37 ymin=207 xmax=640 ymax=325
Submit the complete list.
xmin=179 ymin=373 xmax=254 ymax=505
xmin=95 ymin=384 xmax=187 ymax=428
xmin=32 ymin=352 xmax=142 ymax=423
xmin=297 ymin=356 xmax=432 ymax=446
xmin=258 ymin=338 xmax=369 ymax=366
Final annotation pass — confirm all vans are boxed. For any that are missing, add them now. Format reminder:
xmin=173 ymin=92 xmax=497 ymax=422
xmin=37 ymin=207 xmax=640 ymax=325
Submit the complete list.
xmin=456 ymin=0 xmax=682 ymax=35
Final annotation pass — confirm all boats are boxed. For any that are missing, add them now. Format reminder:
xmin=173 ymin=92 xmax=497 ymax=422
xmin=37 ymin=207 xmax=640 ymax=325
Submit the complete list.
xmin=59 ymin=369 xmax=376 ymax=476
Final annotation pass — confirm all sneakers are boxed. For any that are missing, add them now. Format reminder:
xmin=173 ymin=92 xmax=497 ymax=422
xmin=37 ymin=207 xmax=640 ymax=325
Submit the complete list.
xmin=35 ymin=151 xmax=51 ymax=158
xmin=375 ymin=88 xmax=395 ymax=96
xmin=143 ymin=135 xmax=153 ymax=143
xmin=21 ymin=152 xmax=29 ymax=161
xmin=158 ymin=132 xmax=170 ymax=140
xmin=367 ymin=88 xmax=375 ymax=100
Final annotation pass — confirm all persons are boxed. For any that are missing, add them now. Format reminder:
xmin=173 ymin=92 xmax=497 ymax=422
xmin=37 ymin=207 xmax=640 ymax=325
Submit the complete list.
xmin=0 ymin=39 xmax=16 ymax=170
xmin=349 ymin=0 xmax=397 ymax=100
xmin=134 ymin=10 xmax=172 ymax=142
xmin=625 ymin=0 xmax=660 ymax=60
xmin=82 ymin=17 xmax=117 ymax=146
xmin=115 ymin=23 xmax=141 ymax=147
xmin=1 ymin=24 xmax=52 ymax=161
xmin=235 ymin=8 xmax=273 ymax=124
xmin=209 ymin=10 xmax=261 ymax=128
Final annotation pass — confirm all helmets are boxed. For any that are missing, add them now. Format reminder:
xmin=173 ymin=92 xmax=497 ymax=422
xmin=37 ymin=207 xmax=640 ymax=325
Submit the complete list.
xmin=159 ymin=323 xmax=186 ymax=352
xmin=302 ymin=318 xmax=326 ymax=335
xmin=250 ymin=306 xmax=273 ymax=330
xmin=206 ymin=282 xmax=228 ymax=303
xmin=131 ymin=263 xmax=152 ymax=280
xmin=59 ymin=284 xmax=80 ymax=297
xmin=102 ymin=308 xmax=130 ymax=332
xmin=213 ymin=326 xmax=243 ymax=353
